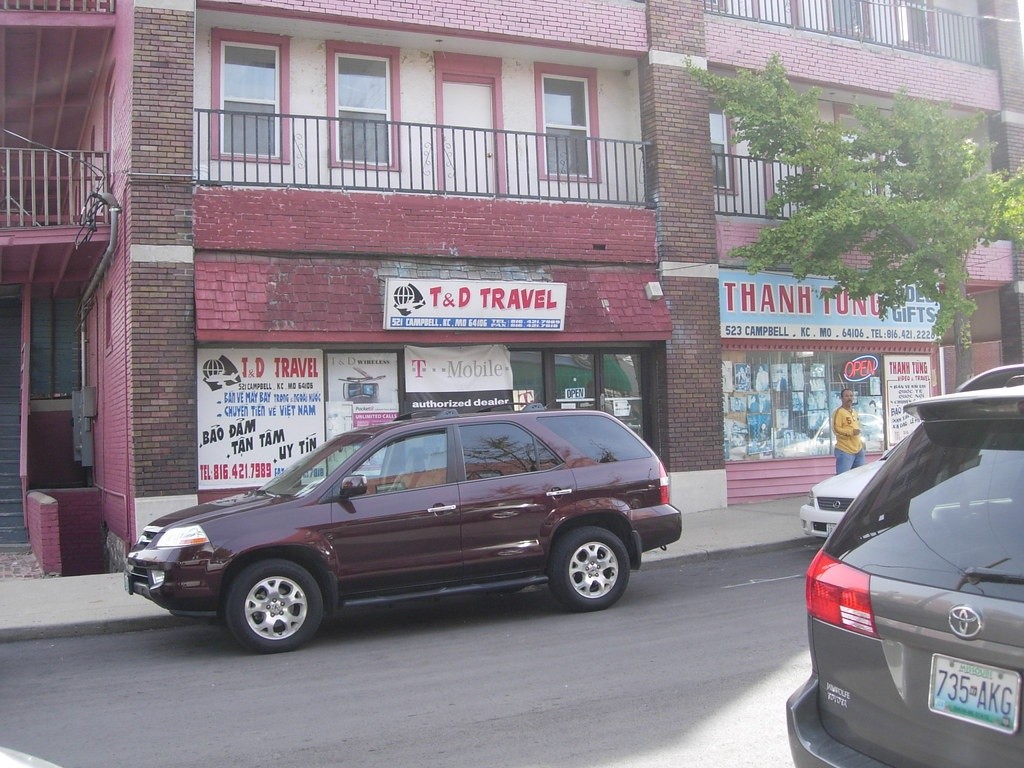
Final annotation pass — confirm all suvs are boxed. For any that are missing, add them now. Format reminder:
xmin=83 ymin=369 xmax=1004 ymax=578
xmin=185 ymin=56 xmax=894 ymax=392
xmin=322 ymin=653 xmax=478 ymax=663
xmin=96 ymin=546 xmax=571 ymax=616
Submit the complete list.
xmin=785 ymin=366 xmax=1023 ymax=768
xmin=122 ymin=408 xmax=683 ymax=652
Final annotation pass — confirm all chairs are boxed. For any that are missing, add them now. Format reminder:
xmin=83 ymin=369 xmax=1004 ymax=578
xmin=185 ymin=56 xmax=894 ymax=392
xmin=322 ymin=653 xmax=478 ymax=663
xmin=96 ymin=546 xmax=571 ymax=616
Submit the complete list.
xmin=392 ymin=446 xmax=448 ymax=493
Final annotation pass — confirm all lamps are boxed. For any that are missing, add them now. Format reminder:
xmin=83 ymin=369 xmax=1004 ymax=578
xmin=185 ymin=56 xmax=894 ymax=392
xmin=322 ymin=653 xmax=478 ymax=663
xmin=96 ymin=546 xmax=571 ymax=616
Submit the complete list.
xmin=644 ymin=280 xmax=663 ymax=302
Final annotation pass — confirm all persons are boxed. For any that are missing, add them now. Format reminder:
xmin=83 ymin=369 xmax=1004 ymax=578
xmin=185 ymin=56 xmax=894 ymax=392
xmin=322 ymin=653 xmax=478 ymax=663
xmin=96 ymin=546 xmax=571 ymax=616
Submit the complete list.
xmin=868 ymin=399 xmax=884 ymax=440
xmin=721 ymin=362 xmax=829 ymax=459
xmin=832 ymin=389 xmax=865 ymax=475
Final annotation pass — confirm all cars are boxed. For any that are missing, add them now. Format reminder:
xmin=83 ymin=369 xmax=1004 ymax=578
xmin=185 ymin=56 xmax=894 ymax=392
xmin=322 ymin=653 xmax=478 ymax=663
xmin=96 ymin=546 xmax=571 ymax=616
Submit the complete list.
xmin=733 ymin=411 xmax=886 ymax=459
xmin=799 ymin=438 xmax=907 ymax=539
